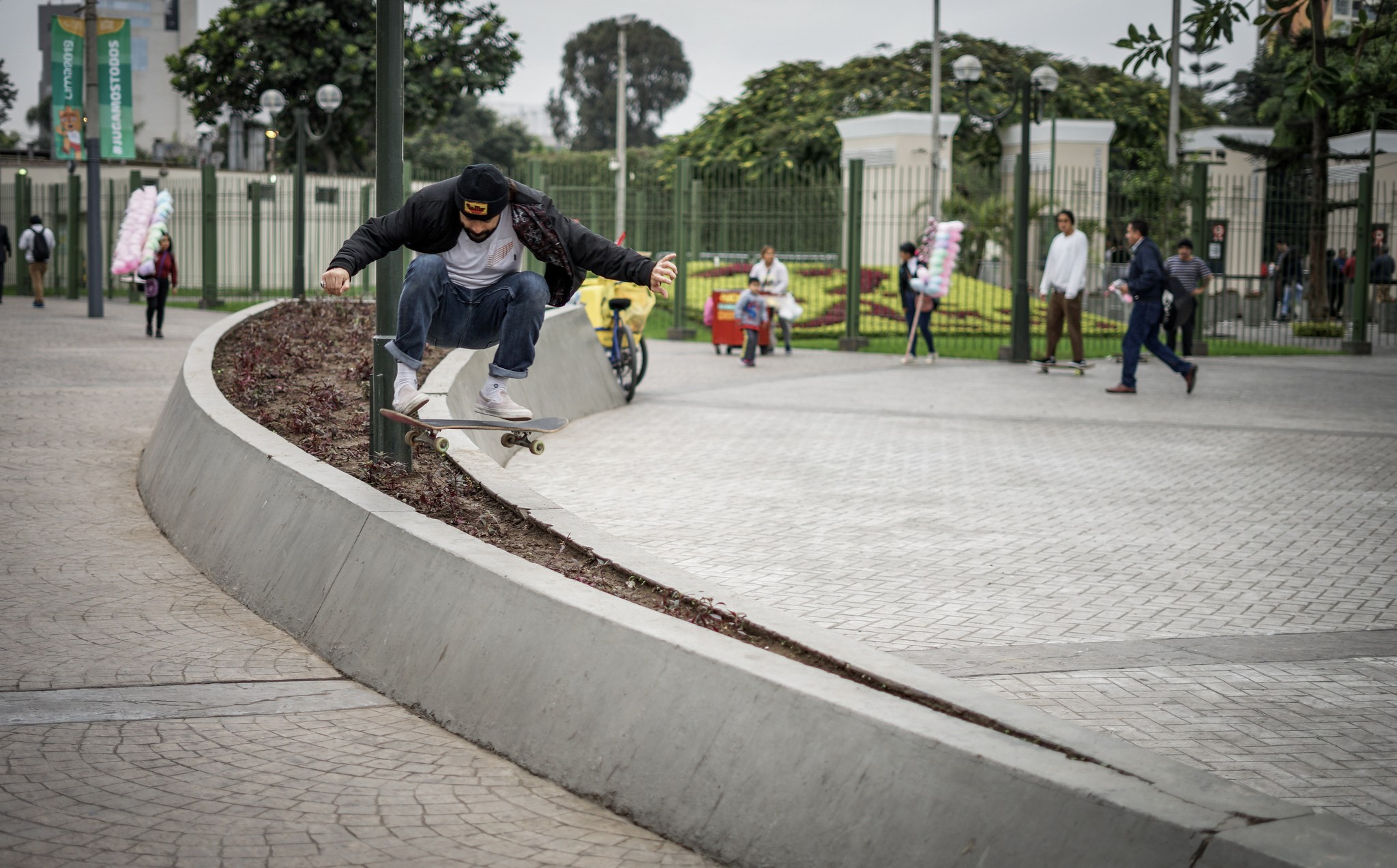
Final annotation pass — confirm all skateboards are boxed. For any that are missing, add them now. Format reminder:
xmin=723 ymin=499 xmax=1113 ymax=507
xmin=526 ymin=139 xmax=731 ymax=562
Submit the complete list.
xmin=379 ymin=408 xmax=570 ymax=455
xmin=1026 ymin=359 xmax=1096 ymax=376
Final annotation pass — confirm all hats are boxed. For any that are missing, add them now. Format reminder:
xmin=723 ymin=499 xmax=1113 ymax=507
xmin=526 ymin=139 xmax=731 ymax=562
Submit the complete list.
xmin=455 ymin=165 xmax=509 ymax=219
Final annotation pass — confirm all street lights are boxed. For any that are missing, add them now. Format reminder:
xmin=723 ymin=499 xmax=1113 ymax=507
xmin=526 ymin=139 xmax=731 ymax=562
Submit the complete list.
xmin=259 ymin=84 xmax=344 ymax=298
xmin=952 ymin=52 xmax=1064 ymax=365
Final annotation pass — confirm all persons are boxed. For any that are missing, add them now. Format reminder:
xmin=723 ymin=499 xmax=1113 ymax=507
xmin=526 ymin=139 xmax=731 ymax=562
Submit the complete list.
xmin=1262 ymin=239 xmax=1303 ymax=324
xmin=1161 ymin=238 xmax=1213 ymax=358
xmin=143 ymin=231 xmax=178 ymax=338
xmin=1032 ymin=208 xmax=1089 ymax=369
xmin=0 ymin=223 xmax=12 ymax=304
xmin=1326 ymin=245 xmax=1395 ymax=323
xmin=897 ymin=240 xmax=940 ymax=365
xmin=1106 ymin=219 xmax=1199 ymax=394
xmin=321 ymin=164 xmax=678 ymax=421
xmin=1106 ymin=242 xmax=1131 ymax=265
xmin=734 ymin=276 xmax=767 ymax=367
xmin=749 ymin=244 xmax=793 ymax=354
xmin=18 ymin=214 xmax=56 ymax=308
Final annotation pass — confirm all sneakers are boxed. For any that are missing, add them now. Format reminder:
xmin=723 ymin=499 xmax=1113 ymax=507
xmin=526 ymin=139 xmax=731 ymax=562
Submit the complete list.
xmin=391 ymin=384 xmax=429 ymax=415
xmin=474 ymin=389 xmax=533 ymax=421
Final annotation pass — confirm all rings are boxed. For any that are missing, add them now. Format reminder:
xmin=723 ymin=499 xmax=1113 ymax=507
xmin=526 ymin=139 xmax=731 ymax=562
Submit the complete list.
xmin=320 ymin=280 xmax=327 ymax=288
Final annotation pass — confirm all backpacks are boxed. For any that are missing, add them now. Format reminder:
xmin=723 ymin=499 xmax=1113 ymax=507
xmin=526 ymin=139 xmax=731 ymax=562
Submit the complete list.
xmin=28 ymin=225 xmax=50 ymax=264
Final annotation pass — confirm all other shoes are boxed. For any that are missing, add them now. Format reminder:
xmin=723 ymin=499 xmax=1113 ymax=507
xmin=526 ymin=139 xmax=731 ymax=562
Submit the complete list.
xmin=1069 ymin=360 xmax=1086 ymax=367
xmin=32 ymin=299 xmax=45 ymax=307
xmin=739 ymin=356 xmax=755 ymax=368
xmin=924 ymin=353 xmax=939 ymax=364
xmin=901 ymin=354 xmax=917 ymax=362
xmin=1033 ymin=357 xmax=1057 ymax=366
xmin=1106 ymin=381 xmax=1138 ymax=395
xmin=1183 ymin=362 xmax=1198 ymax=393
xmin=146 ymin=325 xmax=153 ymax=336
xmin=155 ymin=329 xmax=164 ymax=338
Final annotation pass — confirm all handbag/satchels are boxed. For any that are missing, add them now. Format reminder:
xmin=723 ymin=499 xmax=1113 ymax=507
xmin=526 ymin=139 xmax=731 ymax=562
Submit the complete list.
xmin=137 ymin=276 xmax=159 ymax=293
xmin=779 ymin=292 xmax=804 ymax=322
xmin=1164 ymin=274 xmax=1194 ymax=332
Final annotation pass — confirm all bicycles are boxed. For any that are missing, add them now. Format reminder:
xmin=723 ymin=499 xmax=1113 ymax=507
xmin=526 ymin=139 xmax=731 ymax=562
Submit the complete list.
xmin=591 ymin=295 xmax=650 ymax=404
